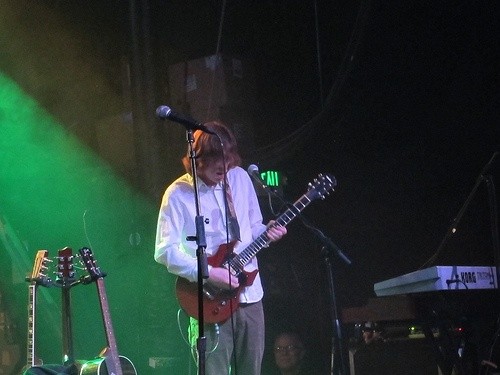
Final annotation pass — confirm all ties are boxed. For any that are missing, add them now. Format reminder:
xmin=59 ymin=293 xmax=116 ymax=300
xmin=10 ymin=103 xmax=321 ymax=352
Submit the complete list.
xmin=222 ymin=175 xmax=240 ymax=241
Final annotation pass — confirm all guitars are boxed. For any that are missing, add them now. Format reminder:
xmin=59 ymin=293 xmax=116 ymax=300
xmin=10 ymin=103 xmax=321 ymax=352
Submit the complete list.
xmin=16 ymin=250 xmax=53 ymax=375
xmin=75 ymin=247 xmax=137 ymax=375
xmin=54 ymin=247 xmax=87 ymax=375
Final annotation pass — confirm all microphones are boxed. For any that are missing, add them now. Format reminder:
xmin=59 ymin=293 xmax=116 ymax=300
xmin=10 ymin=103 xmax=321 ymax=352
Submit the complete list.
xmin=155 ymin=105 xmax=207 ymax=131
xmin=247 ymin=163 xmax=271 ymax=194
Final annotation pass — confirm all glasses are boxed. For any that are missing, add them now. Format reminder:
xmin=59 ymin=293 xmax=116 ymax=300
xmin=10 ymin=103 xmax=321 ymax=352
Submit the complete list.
xmin=273 ymin=345 xmax=304 ymax=352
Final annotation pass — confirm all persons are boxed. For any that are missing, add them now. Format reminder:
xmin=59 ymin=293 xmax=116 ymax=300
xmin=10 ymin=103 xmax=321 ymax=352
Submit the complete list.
xmin=273 ymin=330 xmax=314 ymax=375
xmin=154 ymin=121 xmax=287 ymax=375
xmin=351 ymin=321 xmax=398 ymax=375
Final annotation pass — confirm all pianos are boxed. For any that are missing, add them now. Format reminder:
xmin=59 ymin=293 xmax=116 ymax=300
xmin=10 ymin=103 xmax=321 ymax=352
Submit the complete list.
xmin=373 ymin=266 xmax=497 ymax=375
xmin=174 ymin=173 xmax=336 ymax=325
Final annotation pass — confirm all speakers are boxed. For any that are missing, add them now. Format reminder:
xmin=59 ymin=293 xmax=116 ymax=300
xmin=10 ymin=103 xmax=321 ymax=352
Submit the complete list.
xmin=346 ymin=333 xmax=477 ymax=375
